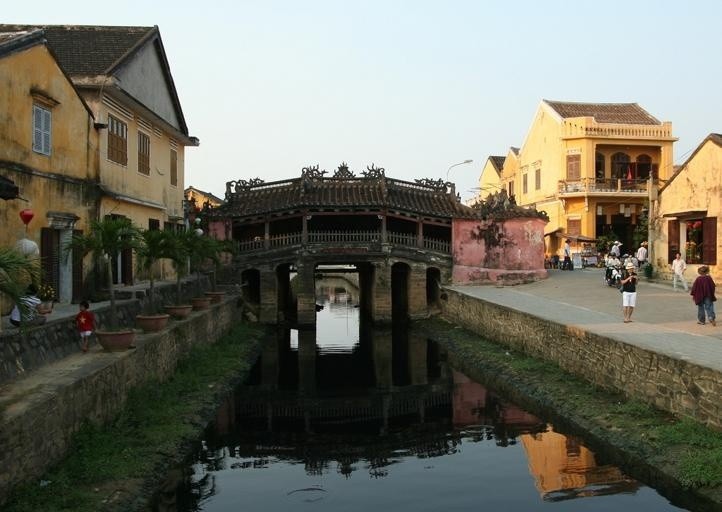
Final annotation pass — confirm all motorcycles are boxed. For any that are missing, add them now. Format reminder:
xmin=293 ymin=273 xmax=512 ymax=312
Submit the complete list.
xmin=605 ymin=265 xmax=626 ymax=289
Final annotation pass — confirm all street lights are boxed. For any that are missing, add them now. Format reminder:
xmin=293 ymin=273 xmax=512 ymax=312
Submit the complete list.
xmin=446 ymin=159 xmax=473 ymax=182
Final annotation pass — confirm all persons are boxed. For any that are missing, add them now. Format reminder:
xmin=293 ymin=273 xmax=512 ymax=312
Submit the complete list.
xmin=10 ymin=283 xmax=51 ymax=327
xmin=689 ymin=267 xmax=717 ymax=328
xmin=74 ymin=302 xmax=99 ymax=351
xmin=672 ymin=252 xmax=689 ymax=292
xmin=561 ymin=238 xmax=574 ymax=270
xmin=603 ymin=241 xmax=647 ymax=322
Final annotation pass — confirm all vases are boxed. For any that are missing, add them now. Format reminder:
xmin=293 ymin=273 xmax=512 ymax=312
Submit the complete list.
xmin=39 ymin=302 xmax=53 ymax=313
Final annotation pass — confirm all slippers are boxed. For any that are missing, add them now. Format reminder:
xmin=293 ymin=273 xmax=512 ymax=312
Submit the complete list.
xmin=624 ymin=320 xmax=632 ymax=323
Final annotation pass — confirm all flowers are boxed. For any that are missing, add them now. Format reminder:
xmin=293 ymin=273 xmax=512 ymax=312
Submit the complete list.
xmin=37 ymin=286 xmax=56 ymax=301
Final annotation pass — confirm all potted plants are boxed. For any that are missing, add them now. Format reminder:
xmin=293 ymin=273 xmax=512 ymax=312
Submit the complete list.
xmin=63 ymin=216 xmax=239 ymax=351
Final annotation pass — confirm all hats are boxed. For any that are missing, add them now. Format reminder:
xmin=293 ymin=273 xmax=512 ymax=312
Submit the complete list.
xmin=698 ymin=266 xmax=710 ymax=275
xmin=625 ymin=263 xmax=635 ymax=269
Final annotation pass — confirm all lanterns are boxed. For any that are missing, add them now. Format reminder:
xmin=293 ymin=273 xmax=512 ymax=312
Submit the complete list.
xmin=14 ymin=201 xmax=39 ymax=257
xmin=192 ymin=204 xmax=206 ymax=236
xmin=686 ymin=221 xmax=702 ymax=229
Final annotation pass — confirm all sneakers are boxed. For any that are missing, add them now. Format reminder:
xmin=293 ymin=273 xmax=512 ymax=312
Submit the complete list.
xmin=710 ymin=320 xmax=716 ymax=327
xmin=697 ymin=322 xmax=705 ymax=325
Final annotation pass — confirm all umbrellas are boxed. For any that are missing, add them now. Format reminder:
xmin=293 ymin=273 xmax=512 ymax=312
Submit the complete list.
xmin=562 ymin=234 xmax=603 ymax=253
